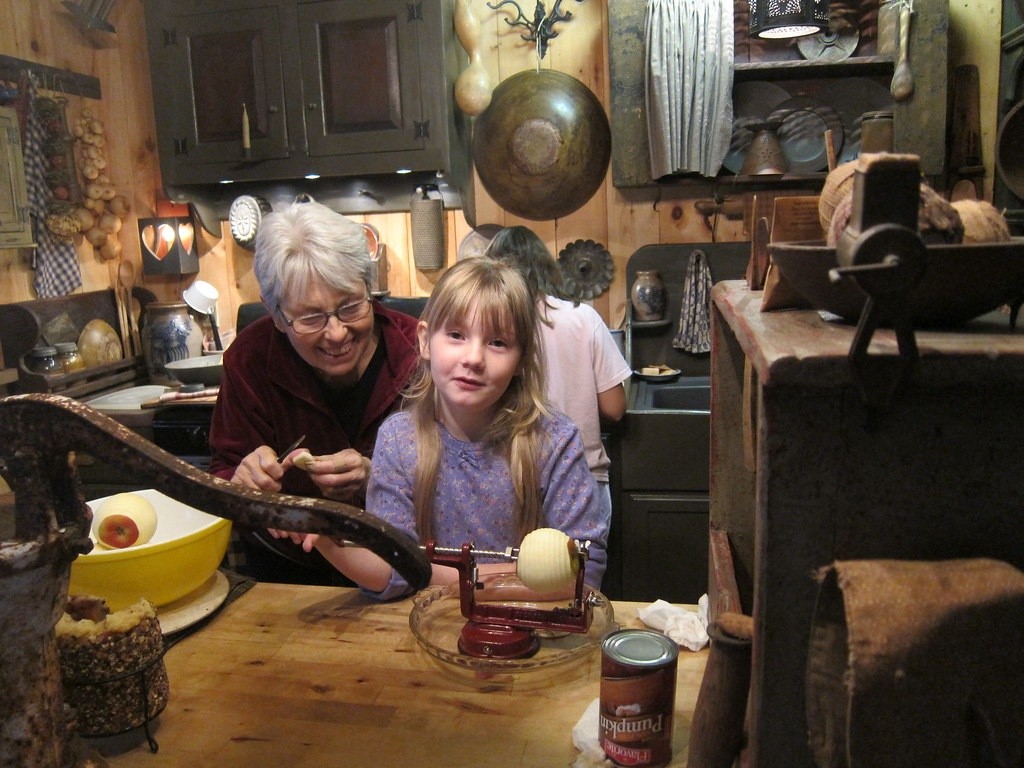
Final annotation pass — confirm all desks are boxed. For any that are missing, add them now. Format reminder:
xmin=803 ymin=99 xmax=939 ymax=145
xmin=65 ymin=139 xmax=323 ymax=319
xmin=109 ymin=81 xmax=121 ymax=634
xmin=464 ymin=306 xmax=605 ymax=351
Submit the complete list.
xmin=69 ymin=581 xmax=746 ymax=768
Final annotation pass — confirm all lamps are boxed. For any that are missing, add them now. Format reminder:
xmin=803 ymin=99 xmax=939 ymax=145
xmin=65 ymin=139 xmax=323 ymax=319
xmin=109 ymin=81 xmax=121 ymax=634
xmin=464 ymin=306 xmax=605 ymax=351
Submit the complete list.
xmin=747 ymin=0 xmax=831 ymax=43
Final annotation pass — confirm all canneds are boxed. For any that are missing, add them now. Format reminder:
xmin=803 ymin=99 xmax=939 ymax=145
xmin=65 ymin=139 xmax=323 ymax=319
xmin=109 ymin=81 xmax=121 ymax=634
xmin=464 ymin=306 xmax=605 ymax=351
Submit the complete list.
xmin=32 ymin=342 xmax=87 ymax=391
xmin=598 ymin=628 xmax=679 ymax=766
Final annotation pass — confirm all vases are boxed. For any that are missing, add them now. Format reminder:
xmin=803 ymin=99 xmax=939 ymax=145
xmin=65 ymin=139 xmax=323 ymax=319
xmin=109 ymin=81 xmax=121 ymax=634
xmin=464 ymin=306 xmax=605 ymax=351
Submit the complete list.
xmin=141 ymin=301 xmax=201 ymax=389
xmin=631 ymin=269 xmax=665 ymax=322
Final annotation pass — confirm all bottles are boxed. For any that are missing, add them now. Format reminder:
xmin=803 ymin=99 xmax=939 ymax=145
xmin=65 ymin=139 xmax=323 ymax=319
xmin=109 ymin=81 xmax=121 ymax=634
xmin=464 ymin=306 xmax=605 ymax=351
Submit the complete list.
xmin=53 ymin=343 xmax=88 ymax=389
xmin=27 ymin=347 xmax=68 ymax=395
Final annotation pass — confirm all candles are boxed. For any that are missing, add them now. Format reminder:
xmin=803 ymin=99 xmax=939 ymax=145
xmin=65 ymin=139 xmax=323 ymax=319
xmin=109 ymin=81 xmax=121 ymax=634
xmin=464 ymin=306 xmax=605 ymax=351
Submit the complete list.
xmin=242 ymin=103 xmax=250 ymax=149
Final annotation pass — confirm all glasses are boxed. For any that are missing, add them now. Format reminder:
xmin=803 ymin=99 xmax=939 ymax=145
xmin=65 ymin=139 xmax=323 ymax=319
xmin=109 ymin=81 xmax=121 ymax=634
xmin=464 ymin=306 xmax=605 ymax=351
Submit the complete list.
xmin=275 ymin=282 xmax=372 ymax=334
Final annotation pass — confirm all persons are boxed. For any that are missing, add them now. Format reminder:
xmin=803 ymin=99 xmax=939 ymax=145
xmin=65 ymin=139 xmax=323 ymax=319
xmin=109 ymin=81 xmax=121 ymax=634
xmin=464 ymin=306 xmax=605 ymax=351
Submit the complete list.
xmin=208 ymin=201 xmax=423 ymax=588
xmin=270 ymin=255 xmax=613 ymax=593
xmin=484 ymin=228 xmax=634 ymax=526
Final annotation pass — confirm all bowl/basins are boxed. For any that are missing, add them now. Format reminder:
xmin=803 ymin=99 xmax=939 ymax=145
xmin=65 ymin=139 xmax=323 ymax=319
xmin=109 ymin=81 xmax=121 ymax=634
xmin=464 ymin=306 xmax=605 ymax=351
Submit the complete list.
xmin=75 ymin=319 xmax=123 ymax=378
xmin=183 ymin=280 xmax=219 ymax=314
xmin=767 ymin=217 xmax=1023 ymax=336
xmin=471 ymin=68 xmax=613 ymax=221
xmin=164 ymin=352 xmax=225 ymax=387
xmin=634 ymin=368 xmax=681 ymax=381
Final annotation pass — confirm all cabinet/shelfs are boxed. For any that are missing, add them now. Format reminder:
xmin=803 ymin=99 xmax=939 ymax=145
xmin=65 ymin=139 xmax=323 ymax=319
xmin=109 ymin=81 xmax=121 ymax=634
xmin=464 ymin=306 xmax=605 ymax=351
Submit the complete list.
xmin=140 ymin=1 xmax=452 ymax=190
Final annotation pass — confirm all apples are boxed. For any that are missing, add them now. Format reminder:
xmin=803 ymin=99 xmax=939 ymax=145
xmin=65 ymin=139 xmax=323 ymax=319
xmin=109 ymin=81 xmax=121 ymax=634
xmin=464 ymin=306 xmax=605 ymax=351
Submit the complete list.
xmin=94 ymin=493 xmax=157 ymax=550
xmin=516 ymin=528 xmax=581 ymax=589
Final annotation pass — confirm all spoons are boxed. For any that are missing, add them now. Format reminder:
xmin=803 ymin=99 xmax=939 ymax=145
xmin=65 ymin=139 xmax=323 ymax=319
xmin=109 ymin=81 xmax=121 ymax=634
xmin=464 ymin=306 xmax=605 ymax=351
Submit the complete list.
xmin=118 ymin=260 xmax=143 ymax=357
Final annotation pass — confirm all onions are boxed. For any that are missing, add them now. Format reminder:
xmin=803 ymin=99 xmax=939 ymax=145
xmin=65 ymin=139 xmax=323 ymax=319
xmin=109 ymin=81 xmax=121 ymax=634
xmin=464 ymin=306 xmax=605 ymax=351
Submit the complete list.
xmin=72 ymin=108 xmax=131 ymax=259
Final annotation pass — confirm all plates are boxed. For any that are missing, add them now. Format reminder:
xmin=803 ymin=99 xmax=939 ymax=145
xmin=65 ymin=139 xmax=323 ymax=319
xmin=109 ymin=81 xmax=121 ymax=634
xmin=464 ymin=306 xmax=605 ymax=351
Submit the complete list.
xmin=154 ymin=568 xmax=229 ymax=636
xmin=60 ymin=490 xmax=235 ymax=617
xmin=766 ymin=93 xmax=843 ymax=170
